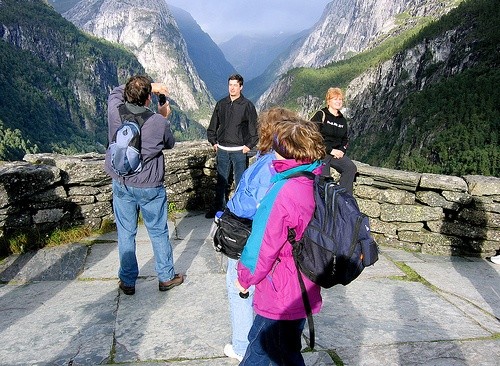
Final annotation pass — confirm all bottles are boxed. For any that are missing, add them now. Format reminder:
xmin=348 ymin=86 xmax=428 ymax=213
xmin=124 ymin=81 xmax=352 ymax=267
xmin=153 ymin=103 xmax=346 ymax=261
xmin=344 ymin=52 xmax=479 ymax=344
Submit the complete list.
xmin=233 ymin=293 xmax=252 ymax=342
xmin=210 ymin=211 xmax=223 ymax=237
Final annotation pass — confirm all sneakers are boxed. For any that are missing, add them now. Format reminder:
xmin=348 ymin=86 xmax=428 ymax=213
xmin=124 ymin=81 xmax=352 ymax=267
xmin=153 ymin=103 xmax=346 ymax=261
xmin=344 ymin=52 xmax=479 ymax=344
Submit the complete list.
xmin=159 ymin=274 xmax=183 ymax=291
xmin=224 ymin=343 xmax=244 ymax=362
xmin=206 ymin=208 xmax=223 ymax=218
xmin=118 ymin=281 xmax=135 ymax=295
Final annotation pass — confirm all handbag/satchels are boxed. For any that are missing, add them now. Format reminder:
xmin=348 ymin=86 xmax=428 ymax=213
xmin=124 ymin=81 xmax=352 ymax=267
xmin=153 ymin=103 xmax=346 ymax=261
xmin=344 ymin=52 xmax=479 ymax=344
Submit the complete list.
xmin=213 ymin=208 xmax=253 ymax=260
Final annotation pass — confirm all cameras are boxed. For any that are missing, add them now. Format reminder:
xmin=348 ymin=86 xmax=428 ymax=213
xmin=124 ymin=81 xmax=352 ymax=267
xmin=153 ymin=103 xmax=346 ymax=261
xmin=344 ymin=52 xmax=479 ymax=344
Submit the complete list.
xmin=159 ymin=94 xmax=166 ymax=106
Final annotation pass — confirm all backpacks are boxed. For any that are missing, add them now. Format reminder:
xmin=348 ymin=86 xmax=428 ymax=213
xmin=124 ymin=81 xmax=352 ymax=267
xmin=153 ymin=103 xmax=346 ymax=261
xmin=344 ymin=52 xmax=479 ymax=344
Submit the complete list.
xmin=108 ymin=104 xmax=155 ymax=178
xmin=281 ymin=161 xmax=379 ymax=289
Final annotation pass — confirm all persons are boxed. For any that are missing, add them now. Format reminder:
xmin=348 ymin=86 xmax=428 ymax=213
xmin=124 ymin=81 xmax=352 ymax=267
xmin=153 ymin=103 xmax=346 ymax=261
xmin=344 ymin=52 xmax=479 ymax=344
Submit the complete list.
xmin=205 ymin=74 xmax=261 ymax=219
xmin=214 ymin=107 xmax=302 ymax=361
xmin=235 ymin=116 xmax=329 ymax=366
xmin=307 ymin=86 xmax=356 ymax=199
xmin=103 ymin=76 xmax=186 ymax=296
